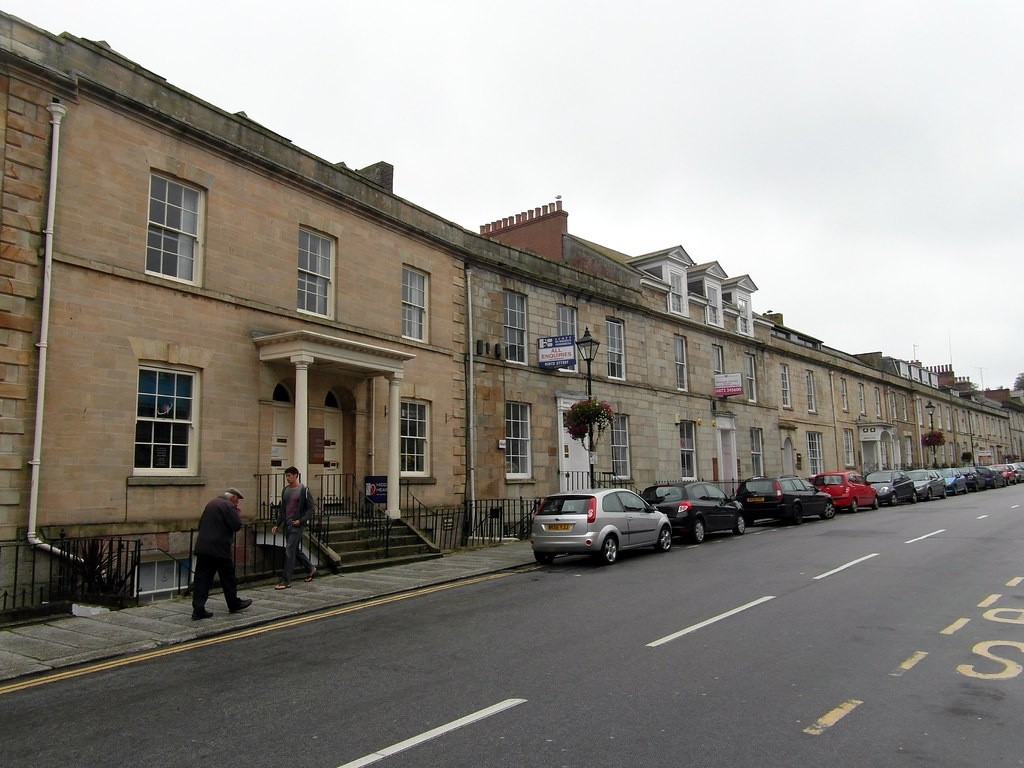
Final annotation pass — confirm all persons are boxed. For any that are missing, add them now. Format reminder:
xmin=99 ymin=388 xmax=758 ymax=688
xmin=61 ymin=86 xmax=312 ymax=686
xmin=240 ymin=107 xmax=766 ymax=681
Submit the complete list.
xmin=191 ymin=487 xmax=252 ymax=620
xmin=272 ymin=466 xmax=316 ymax=589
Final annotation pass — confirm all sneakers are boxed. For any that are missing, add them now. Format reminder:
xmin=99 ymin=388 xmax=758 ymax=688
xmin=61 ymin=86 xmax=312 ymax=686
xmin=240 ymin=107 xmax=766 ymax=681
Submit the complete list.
xmin=228 ymin=598 xmax=252 ymax=614
xmin=192 ymin=609 xmax=213 ymax=620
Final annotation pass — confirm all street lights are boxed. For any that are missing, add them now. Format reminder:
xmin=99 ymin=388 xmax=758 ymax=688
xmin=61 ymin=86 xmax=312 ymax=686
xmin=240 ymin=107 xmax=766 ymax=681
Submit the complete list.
xmin=924 ymin=400 xmax=938 ymax=464
xmin=575 ymin=325 xmax=602 ymax=490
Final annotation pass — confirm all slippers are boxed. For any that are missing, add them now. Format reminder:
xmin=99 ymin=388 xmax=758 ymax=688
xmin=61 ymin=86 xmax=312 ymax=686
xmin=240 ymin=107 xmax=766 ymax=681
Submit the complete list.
xmin=275 ymin=582 xmax=291 ymax=590
xmin=304 ymin=568 xmax=316 ymax=582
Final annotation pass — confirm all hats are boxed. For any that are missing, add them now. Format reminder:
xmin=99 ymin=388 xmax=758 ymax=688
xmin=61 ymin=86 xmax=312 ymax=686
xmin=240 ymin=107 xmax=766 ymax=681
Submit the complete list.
xmin=225 ymin=487 xmax=243 ymax=499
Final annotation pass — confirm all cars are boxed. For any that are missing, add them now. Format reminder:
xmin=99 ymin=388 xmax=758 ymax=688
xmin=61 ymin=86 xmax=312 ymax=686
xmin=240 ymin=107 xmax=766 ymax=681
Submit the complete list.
xmin=641 ymin=480 xmax=746 ymax=543
xmin=906 ymin=469 xmax=947 ymax=502
xmin=957 ymin=462 xmax=1024 ymax=492
xmin=530 ymin=488 xmax=673 ymax=567
xmin=812 ymin=470 xmax=879 ymax=513
xmin=735 ymin=474 xmax=835 ymax=527
xmin=866 ymin=470 xmax=917 ymax=506
xmin=937 ymin=468 xmax=968 ymax=496
